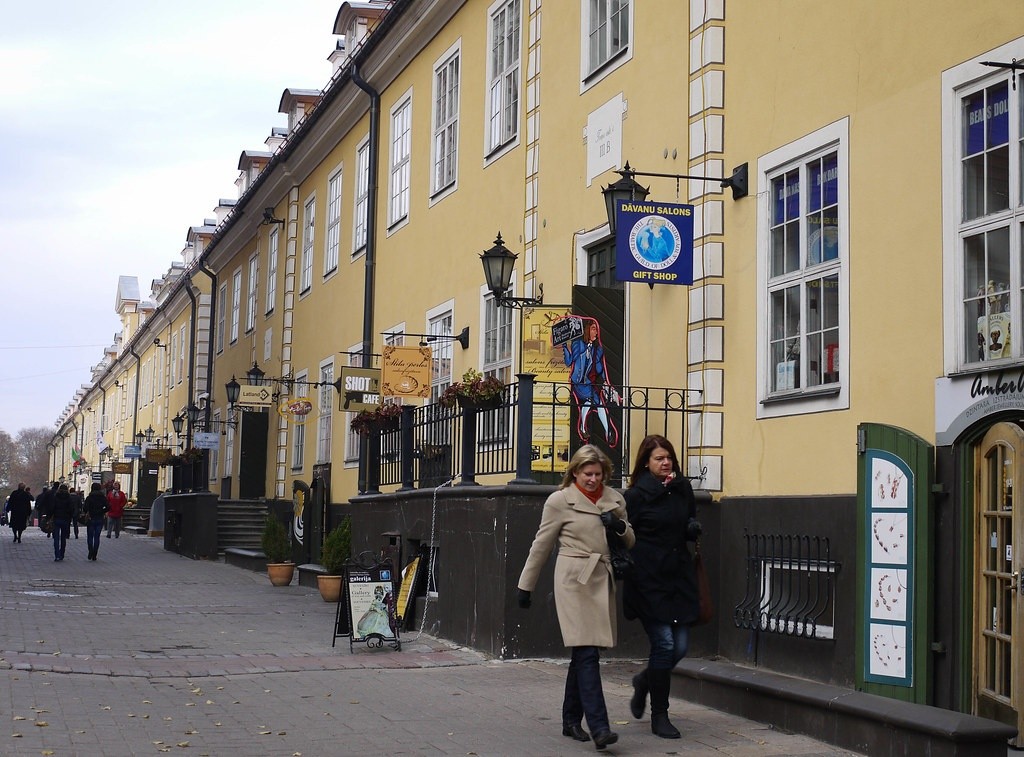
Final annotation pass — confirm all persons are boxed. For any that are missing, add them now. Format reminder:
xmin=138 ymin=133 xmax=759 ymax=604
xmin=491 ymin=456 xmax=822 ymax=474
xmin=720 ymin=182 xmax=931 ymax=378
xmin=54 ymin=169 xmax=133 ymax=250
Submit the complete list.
xmin=622 ymin=435 xmax=700 ymax=739
xmin=34 ymin=481 xmax=82 ymax=562
xmin=6 ymin=482 xmax=35 ymax=543
xmin=513 ymin=443 xmax=636 ymax=750
xmin=83 ymin=482 xmax=111 ymax=561
xmin=105 ymin=481 xmax=127 ymax=538
xmin=4 ymin=500 xmax=11 ymax=523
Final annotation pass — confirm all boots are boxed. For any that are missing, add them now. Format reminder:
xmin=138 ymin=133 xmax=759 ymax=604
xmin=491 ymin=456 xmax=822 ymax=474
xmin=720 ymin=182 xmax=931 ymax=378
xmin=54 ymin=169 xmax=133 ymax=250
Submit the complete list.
xmin=631 ymin=668 xmax=648 ymax=719
xmin=646 ymin=669 xmax=681 ymax=739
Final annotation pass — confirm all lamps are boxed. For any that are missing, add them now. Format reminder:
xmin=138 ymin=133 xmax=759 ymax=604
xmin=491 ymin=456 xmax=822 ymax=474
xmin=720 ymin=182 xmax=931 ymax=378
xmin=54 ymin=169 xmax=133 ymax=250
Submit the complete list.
xmin=245 ymin=360 xmax=278 ymax=405
xmin=153 ymin=338 xmax=166 ymax=351
xmin=171 ymin=410 xmax=187 ymax=439
xmin=135 ymin=428 xmax=145 ymax=446
xmin=185 ymin=403 xmax=211 ymax=432
xmin=87 ymin=407 xmax=95 ymax=413
xmin=599 ymin=160 xmax=750 ymax=239
xmin=115 ymin=379 xmax=123 ymax=389
xmin=476 ymin=230 xmax=545 ymax=308
xmin=262 ymin=207 xmax=285 ymax=230
xmin=106 ymin=444 xmax=118 ymax=462
xmin=226 ymin=374 xmax=255 ymax=413
xmin=144 ymin=424 xmax=155 ymax=442
xmin=64 ymin=433 xmax=70 ymax=437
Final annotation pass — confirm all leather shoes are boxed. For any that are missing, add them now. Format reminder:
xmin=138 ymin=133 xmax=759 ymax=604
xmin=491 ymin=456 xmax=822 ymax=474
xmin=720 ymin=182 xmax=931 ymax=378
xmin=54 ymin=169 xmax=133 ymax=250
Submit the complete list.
xmin=593 ymin=728 xmax=618 ymax=750
xmin=563 ymin=721 xmax=591 ymax=742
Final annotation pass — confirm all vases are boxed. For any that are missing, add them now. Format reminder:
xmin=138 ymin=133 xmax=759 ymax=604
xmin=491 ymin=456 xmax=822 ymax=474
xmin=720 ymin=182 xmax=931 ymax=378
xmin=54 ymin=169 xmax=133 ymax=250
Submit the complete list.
xmin=368 ymin=416 xmax=396 ymax=431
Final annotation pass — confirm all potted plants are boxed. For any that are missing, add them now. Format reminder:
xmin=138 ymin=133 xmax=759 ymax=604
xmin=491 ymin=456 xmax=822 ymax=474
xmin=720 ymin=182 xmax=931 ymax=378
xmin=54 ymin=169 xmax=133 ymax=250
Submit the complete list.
xmin=316 ymin=510 xmax=360 ymax=603
xmin=258 ymin=496 xmax=296 ymax=586
xmin=456 ymin=389 xmax=503 ymax=410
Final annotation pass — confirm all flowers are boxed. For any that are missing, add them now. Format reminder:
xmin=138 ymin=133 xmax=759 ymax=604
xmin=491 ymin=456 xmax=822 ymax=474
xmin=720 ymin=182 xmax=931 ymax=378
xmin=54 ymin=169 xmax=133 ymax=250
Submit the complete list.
xmin=438 ymin=367 xmax=505 ymax=408
xmin=349 ymin=400 xmax=401 ymax=432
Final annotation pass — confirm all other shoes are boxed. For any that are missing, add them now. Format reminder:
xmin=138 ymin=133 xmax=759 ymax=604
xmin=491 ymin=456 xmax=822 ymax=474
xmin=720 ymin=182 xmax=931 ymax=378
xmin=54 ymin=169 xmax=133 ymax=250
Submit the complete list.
xmin=106 ymin=535 xmax=111 ymax=538
xmin=88 ymin=553 xmax=92 ymax=559
xmin=13 ymin=538 xmax=16 ymax=542
xmin=115 ymin=536 xmax=118 ymax=538
xmin=91 ymin=554 xmax=97 ymax=560
xmin=18 ymin=539 xmax=21 ymax=543
xmin=75 ymin=536 xmax=78 ymax=539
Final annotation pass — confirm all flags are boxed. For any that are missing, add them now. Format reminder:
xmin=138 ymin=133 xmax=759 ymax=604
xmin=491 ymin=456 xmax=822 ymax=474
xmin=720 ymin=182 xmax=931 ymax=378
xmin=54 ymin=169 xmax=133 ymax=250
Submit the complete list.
xmin=72 ymin=445 xmax=85 ymax=467
xmin=96 ymin=429 xmax=109 ymax=455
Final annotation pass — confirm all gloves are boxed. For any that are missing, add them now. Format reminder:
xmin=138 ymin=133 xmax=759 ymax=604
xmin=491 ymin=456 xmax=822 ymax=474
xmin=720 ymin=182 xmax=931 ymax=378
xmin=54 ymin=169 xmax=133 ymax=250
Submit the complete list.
xmin=600 ymin=512 xmax=626 ymax=533
xmin=518 ymin=590 xmax=532 ymax=609
xmin=687 ymin=521 xmax=702 ymax=539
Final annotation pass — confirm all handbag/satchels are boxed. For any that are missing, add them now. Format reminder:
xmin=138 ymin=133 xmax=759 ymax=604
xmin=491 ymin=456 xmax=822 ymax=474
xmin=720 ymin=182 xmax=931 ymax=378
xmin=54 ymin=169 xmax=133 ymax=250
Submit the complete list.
xmin=77 ymin=505 xmax=89 ymax=525
xmin=38 ymin=516 xmax=54 ymax=533
xmin=690 ymin=538 xmax=715 ymax=629
xmin=606 ymin=524 xmax=637 ymax=580
xmin=0 ymin=512 xmax=8 ymax=526
